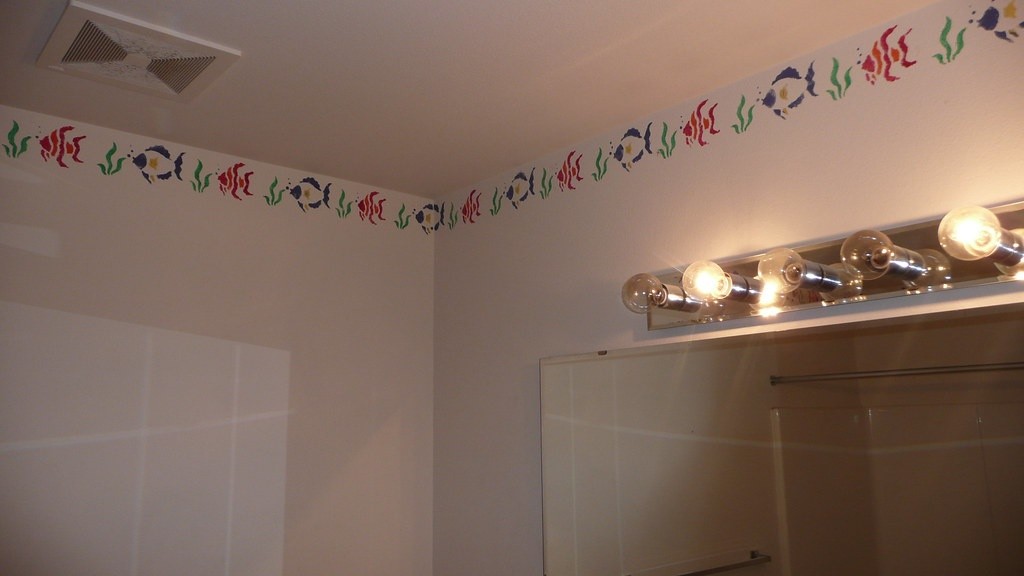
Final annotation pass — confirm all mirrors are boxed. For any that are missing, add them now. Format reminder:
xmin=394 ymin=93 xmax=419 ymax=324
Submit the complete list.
xmin=538 ymin=302 xmax=1024 ymax=576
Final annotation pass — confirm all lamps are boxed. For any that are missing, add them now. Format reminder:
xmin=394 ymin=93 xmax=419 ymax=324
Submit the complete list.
xmin=620 ymin=200 xmax=1024 ymax=333
